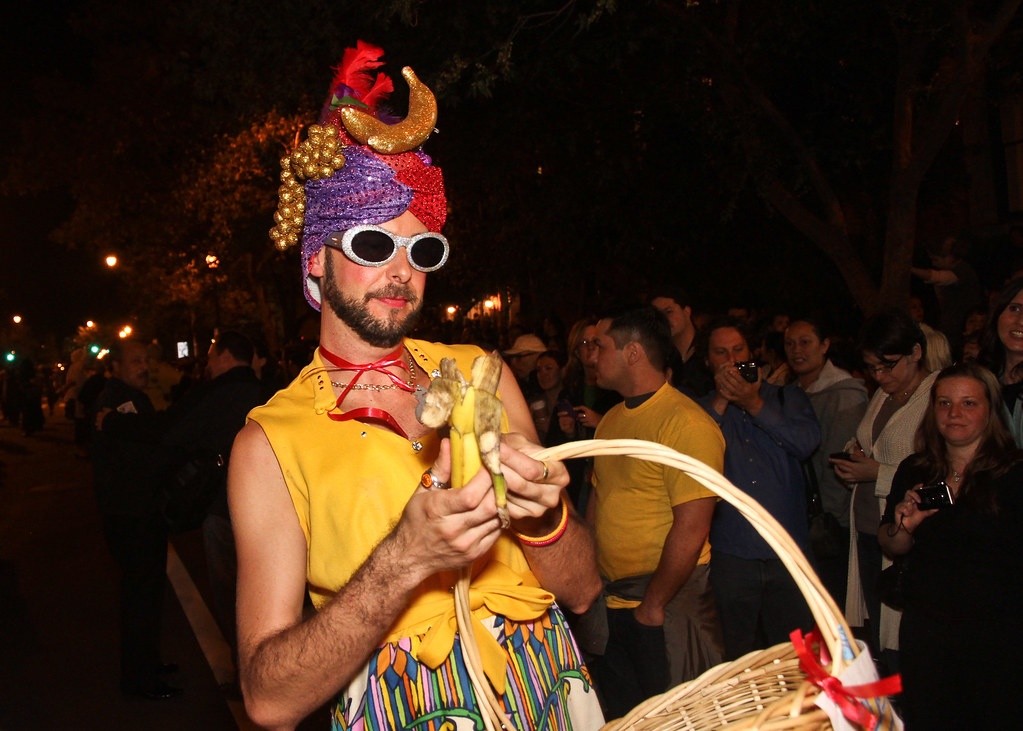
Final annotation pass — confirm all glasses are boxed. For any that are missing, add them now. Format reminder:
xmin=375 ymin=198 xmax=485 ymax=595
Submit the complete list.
xmin=323 ymin=224 xmax=449 ymax=272
xmin=576 ymin=338 xmax=597 ymax=349
xmin=862 ymin=353 xmax=905 ymax=375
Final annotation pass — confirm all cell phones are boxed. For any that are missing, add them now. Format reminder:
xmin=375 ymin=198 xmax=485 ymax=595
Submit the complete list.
xmin=829 ymin=452 xmax=855 ymax=463
xmin=556 ymin=400 xmax=579 ymax=419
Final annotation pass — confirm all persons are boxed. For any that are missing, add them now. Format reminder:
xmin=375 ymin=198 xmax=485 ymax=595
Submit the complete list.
xmin=502 ymin=275 xmax=1023 ymax=717
xmin=227 ymin=143 xmax=607 ymax=731
xmin=876 ymin=362 xmax=1023 ymax=731
xmin=0 ymin=330 xmax=315 ymax=701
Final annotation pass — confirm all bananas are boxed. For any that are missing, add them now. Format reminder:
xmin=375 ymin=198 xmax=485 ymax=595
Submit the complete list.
xmin=413 ymin=350 xmax=508 ymax=528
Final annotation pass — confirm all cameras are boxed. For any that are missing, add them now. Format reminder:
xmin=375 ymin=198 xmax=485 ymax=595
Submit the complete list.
xmin=914 ymin=482 xmax=955 ymax=511
xmin=734 ymin=362 xmax=759 ymax=382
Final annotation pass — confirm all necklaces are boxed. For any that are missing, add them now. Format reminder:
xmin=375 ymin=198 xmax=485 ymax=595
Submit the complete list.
xmin=948 ymin=470 xmax=964 ymax=489
xmin=904 ymin=379 xmax=923 ymax=401
xmin=330 ymin=356 xmax=416 ymax=392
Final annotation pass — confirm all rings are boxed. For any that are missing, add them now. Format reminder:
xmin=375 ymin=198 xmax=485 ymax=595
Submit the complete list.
xmin=583 ymin=414 xmax=587 ymax=418
xmin=422 ymin=469 xmax=445 ymax=490
xmin=539 ymin=461 xmax=548 ymax=484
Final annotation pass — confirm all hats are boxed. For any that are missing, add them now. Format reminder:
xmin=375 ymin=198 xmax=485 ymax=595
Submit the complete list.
xmin=503 ymin=334 xmax=549 ymax=355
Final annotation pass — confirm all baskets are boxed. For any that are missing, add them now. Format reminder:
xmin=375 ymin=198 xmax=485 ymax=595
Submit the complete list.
xmin=451 ymin=437 xmax=905 ymax=731
